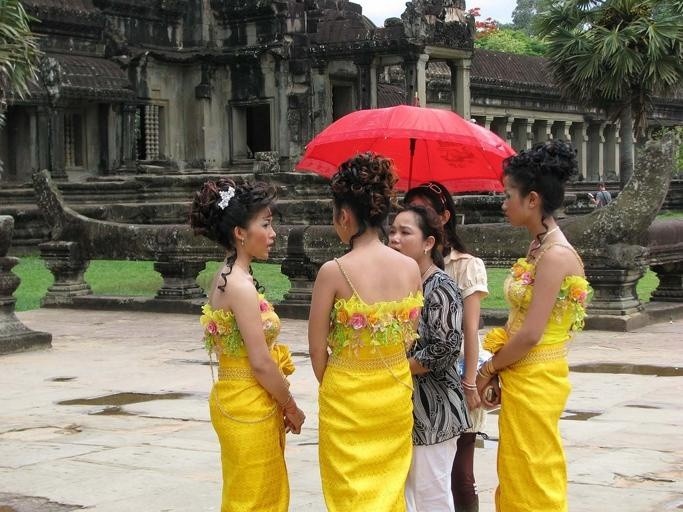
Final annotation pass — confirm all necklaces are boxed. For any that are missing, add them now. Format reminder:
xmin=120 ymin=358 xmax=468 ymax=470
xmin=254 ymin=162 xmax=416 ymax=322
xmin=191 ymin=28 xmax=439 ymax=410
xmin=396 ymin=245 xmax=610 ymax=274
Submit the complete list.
xmin=526 ymin=225 xmax=560 ymax=263
xmin=422 ymin=264 xmax=434 ymax=278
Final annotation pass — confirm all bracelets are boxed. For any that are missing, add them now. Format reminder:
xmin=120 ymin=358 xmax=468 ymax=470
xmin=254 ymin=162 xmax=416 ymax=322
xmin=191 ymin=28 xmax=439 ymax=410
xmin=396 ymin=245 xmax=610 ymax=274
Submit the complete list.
xmin=279 ymin=392 xmax=293 ymax=407
xmin=460 ymin=380 xmax=477 ymax=390
xmin=477 ymin=357 xmax=497 ymax=379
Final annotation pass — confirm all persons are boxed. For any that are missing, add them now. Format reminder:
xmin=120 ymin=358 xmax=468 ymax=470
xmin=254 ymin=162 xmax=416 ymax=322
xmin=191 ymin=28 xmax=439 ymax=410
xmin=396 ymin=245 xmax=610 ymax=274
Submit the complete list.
xmin=589 ymin=183 xmax=612 ymax=210
xmin=402 ymin=181 xmax=489 ymax=512
xmin=475 ymin=137 xmax=590 ymax=512
xmin=190 ymin=172 xmax=306 ymax=512
xmin=387 ymin=202 xmax=473 ymax=512
xmin=307 ymin=151 xmax=425 ymax=512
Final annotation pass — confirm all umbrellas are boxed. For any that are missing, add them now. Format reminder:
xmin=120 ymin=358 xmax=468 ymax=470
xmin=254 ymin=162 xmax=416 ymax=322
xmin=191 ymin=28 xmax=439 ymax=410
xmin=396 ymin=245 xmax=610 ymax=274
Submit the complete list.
xmin=292 ymin=92 xmax=520 ymax=192
